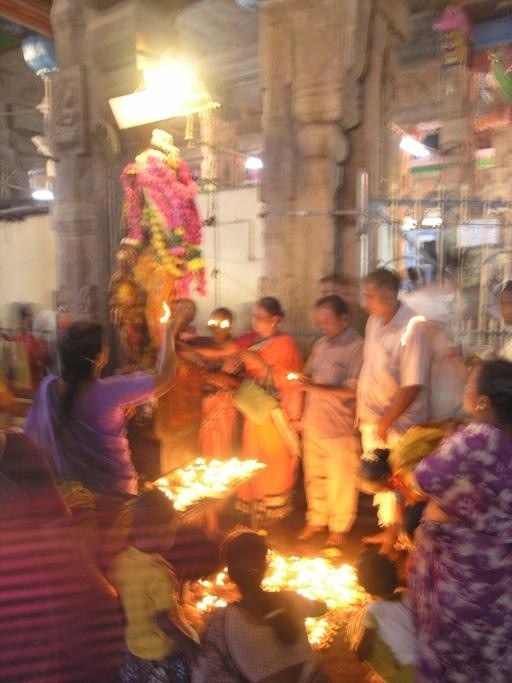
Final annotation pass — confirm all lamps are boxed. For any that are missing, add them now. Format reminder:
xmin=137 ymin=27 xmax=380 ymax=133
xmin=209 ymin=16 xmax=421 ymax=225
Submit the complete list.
xmin=27 ymin=168 xmax=54 ymax=201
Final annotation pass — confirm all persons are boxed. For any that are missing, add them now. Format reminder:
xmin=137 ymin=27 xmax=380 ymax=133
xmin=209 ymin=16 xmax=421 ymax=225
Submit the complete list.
xmin=349 ymin=547 xmax=418 ymax=682
xmin=197 ymin=308 xmax=244 ymax=462
xmin=291 ymin=293 xmax=365 ymax=545
xmin=107 ymin=487 xmax=211 ymax=682
xmin=497 ymin=278 xmax=512 ymax=362
xmin=158 ymin=299 xmax=206 ymax=463
xmin=353 ymin=267 xmax=433 ymax=570
xmin=24 ymin=308 xmax=182 ymax=493
xmin=192 ymin=528 xmax=329 ymax=682
xmin=192 ymin=296 xmax=303 ymax=527
xmin=405 ymin=360 xmax=511 ymax=681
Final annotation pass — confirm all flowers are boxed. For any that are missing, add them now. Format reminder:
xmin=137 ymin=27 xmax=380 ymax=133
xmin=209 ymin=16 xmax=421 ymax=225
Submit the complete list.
xmin=119 ymin=148 xmax=207 ymax=296
